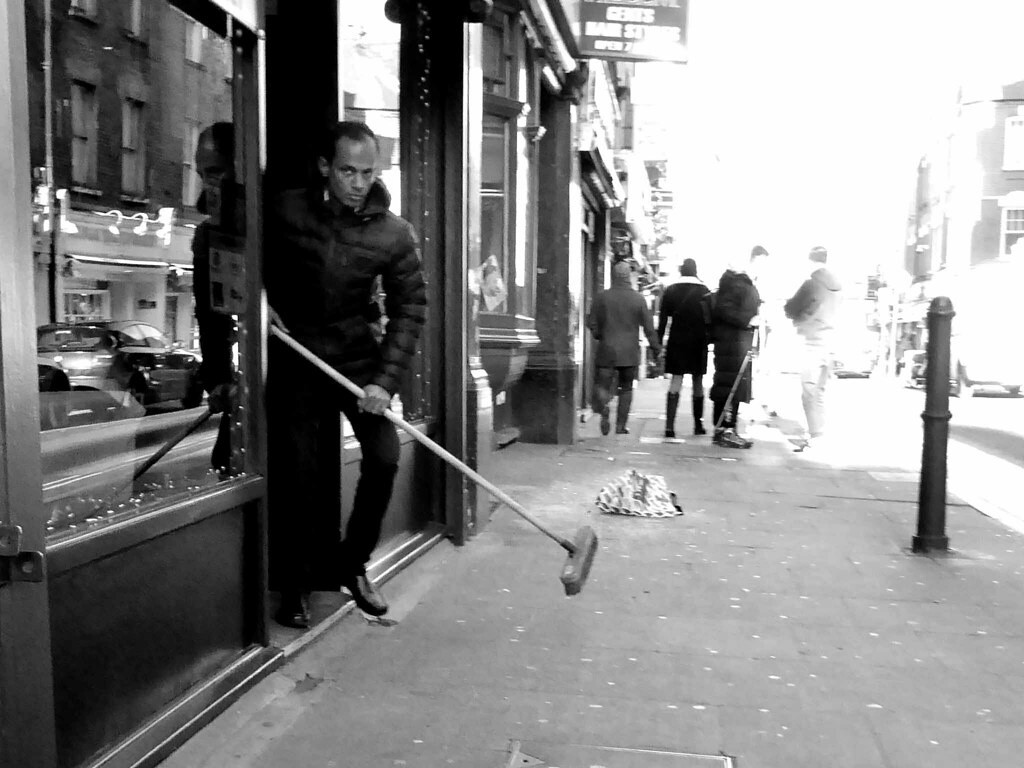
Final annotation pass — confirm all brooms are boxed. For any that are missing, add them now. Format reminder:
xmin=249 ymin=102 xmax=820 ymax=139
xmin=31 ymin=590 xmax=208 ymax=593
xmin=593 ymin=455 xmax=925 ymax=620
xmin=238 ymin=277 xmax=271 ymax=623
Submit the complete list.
xmin=271 ymin=324 xmax=598 ymax=596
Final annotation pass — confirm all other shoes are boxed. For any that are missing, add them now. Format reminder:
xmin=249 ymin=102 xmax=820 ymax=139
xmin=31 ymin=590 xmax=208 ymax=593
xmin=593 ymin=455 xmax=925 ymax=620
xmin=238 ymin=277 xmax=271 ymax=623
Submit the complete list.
xmin=276 ymin=591 xmax=310 ymax=628
xmin=714 ymin=429 xmax=752 ymax=449
xmin=349 ymin=575 xmax=387 ymax=616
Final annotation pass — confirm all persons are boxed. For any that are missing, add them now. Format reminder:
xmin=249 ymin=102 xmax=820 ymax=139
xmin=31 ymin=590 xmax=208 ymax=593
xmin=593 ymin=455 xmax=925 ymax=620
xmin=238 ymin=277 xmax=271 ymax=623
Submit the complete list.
xmin=653 ymin=259 xmax=714 ymax=437
xmin=709 ymin=243 xmax=845 ymax=446
xmin=258 ymin=121 xmax=426 ymax=629
xmin=586 ymin=260 xmax=661 ymax=435
xmin=191 ymin=119 xmax=253 ymax=485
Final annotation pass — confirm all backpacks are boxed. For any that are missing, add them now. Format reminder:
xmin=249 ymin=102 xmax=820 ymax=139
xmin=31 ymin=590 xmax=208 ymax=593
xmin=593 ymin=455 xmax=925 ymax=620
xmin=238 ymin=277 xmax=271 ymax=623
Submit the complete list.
xmin=698 ymin=291 xmax=719 ymax=344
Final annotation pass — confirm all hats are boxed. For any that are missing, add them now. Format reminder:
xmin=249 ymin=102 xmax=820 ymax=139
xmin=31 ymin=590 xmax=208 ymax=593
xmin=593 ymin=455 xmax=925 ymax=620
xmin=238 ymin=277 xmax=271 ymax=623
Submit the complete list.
xmin=611 ymin=261 xmax=632 ymax=284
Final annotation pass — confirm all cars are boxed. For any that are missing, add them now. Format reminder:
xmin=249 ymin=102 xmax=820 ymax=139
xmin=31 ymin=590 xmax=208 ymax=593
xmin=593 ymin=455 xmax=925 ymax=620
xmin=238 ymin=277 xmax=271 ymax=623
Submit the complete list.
xmin=38 ymin=318 xmax=206 ymax=410
xmin=834 ymin=350 xmax=1021 ymax=398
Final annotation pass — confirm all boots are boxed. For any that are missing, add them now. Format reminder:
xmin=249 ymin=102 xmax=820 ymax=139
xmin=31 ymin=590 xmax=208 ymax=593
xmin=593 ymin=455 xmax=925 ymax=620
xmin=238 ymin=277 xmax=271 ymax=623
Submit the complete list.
xmin=616 ymin=391 xmax=631 ymax=434
xmin=665 ymin=392 xmax=679 ymax=437
xmin=693 ymin=395 xmax=706 ymax=435
xmin=601 ymin=405 xmax=610 ymax=435
